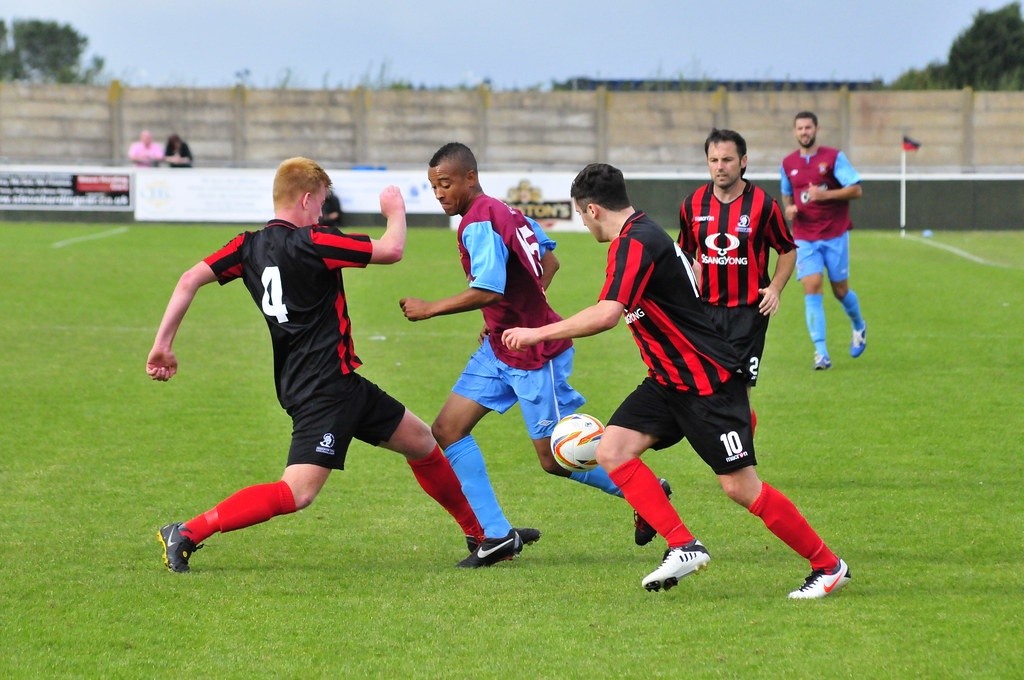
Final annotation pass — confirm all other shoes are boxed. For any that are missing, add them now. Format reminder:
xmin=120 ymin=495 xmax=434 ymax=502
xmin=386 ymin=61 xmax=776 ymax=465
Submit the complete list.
xmin=849 ymin=320 xmax=867 ymax=358
xmin=813 ymin=353 xmax=832 ymax=370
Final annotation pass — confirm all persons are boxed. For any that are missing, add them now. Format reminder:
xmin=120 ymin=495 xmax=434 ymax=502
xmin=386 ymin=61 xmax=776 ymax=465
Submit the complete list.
xmin=164 ymin=135 xmax=193 ymax=167
xmin=398 ymin=140 xmax=673 ymax=568
xmin=145 ymin=155 xmax=541 ymax=575
xmin=778 ymin=110 xmax=867 ymax=371
xmin=318 ymin=192 xmax=343 ymax=226
xmin=128 ymin=130 xmax=164 ymax=167
xmin=501 ymin=164 xmax=852 ymax=601
xmin=677 ymin=125 xmax=802 ymax=440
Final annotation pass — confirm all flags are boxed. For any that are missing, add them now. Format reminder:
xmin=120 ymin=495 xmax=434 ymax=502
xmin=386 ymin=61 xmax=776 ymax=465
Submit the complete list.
xmin=903 ymin=137 xmax=921 ymax=151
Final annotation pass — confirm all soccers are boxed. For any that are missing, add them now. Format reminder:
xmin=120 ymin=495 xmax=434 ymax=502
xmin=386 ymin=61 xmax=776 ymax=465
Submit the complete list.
xmin=550 ymin=412 xmax=605 ymax=474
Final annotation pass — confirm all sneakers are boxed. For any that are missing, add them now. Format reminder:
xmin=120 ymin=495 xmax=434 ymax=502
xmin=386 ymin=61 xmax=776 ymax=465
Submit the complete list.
xmin=786 ymin=558 xmax=853 ymax=600
xmin=641 ymin=538 xmax=712 ymax=592
xmin=465 ymin=527 xmax=543 ymax=554
xmin=156 ymin=521 xmax=197 ymax=575
xmin=634 ymin=477 xmax=673 ymax=546
xmin=453 ymin=528 xmax=524 ymax=570
